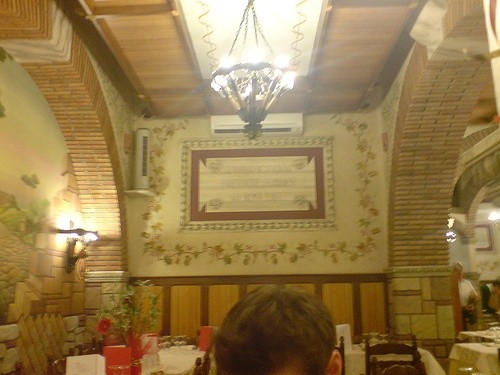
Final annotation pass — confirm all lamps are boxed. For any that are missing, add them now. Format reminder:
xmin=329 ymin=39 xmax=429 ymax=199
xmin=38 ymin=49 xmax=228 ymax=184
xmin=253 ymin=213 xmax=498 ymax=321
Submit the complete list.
xmin=51 ymin=216 xmax=100 ymax=272
xmin=205 ymin=0 xmax=296 ymax=139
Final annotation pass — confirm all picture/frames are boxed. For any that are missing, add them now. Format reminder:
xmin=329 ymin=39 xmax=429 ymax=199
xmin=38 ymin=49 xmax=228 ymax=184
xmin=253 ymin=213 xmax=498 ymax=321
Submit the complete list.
xmin=175 ymin=139 xmax=338 ymax=231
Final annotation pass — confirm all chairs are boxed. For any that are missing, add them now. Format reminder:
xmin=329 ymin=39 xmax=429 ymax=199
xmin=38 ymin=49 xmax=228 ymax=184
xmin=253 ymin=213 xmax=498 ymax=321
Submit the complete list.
xmin=331 ymin=329 xmax=426 ymax=375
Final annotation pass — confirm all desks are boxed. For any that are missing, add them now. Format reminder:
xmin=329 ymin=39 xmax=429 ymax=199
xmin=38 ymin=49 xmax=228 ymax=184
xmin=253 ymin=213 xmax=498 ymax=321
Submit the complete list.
xmin=330 ymin=343 xmax=446 ymax=375
xmin=65 ymin=344 xmax=213 ymax=375
xmin=448 ymin=342 xmax=500 ymax=375
xmin=458 ymin=329 xmax=500 ymax=342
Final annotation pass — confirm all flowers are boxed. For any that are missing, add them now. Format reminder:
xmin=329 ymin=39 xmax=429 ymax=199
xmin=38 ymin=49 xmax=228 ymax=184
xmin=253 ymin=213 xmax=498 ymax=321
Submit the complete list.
xmin=93 ymin=278 xmax=158 ymax=346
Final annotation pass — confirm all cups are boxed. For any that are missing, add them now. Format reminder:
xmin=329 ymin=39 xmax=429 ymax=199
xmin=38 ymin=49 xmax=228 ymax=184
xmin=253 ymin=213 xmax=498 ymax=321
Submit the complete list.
xmin=158 ymin=335 xmax=191 ymax=347
xmin=108 ymin=365 xmax=130 ymax=375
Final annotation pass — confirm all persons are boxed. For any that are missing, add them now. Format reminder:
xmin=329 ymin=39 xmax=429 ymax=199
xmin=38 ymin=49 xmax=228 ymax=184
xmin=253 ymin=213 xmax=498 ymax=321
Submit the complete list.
xmin=480 ymin=280 xmax=500 ymax=314
xmin=214 ymin=284 xmax=342 ymax=375
xmin=451 ymin=261 xmax=478 ymax=331
